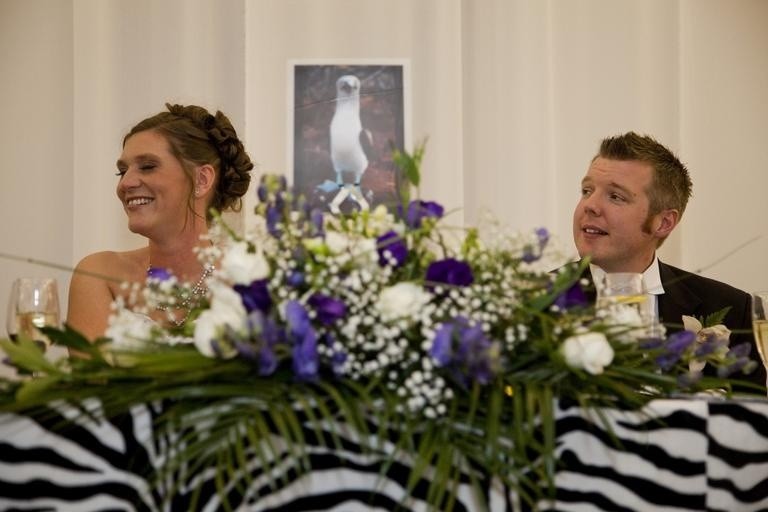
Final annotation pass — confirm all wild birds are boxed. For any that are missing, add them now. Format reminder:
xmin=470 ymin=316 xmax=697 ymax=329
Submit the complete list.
xmin=321 ymin=73 xmax=377 ymax=191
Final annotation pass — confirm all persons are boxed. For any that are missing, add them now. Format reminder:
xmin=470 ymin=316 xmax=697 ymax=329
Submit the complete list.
xmin=545 ymin=131 xmax=766 ymax=393
xmin=66 ymin=103 xmax=256 ymax=391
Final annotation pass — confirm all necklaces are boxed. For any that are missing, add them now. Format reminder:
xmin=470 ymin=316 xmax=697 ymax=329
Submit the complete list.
xmin=145 ymin=234 xmax=219 ymax=329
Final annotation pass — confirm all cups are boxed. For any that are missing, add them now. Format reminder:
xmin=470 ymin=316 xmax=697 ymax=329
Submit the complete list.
xmin=8 ymin=275 xmax=61 ymax=347
xmin=749 ymin=289 xmax=768 ymax=369
xmin=595 ymin=272 xmax=652 ymax=332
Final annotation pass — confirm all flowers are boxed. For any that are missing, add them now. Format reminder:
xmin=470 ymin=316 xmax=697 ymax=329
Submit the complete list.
xmin=1 ymin=129 xmax=762 ymax=512
